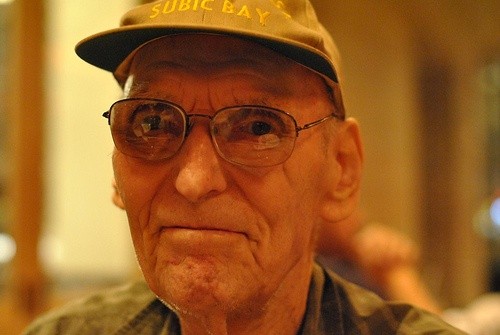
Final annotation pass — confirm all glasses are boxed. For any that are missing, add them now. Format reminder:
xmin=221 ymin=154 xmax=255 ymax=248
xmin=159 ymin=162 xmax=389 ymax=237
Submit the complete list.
xmin=101 ymin=98 xmax=337 ymax=168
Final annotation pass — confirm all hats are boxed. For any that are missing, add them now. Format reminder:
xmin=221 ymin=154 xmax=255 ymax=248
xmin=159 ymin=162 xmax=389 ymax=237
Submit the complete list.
xmin=74 ymin=0 xmax=348 ymax=120
